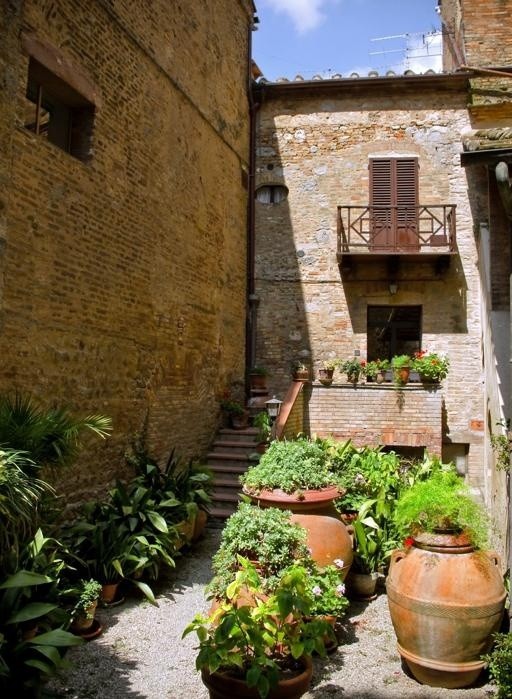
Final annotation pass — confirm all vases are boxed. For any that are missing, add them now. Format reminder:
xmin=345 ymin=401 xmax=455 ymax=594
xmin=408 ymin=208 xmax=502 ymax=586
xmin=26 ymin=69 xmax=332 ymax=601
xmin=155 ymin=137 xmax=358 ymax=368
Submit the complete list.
xmin=374 ymin=369 xmax=387 ymax=384
xmin=419 ymin=371 xmax=432 ymax=381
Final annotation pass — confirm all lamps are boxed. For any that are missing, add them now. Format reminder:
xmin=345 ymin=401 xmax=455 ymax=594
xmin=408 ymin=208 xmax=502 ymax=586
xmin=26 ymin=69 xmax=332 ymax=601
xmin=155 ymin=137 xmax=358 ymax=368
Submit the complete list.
xmin=388 ymin=282 xmax=400 ymax=295
xmin=263 ymin=394 xmax=283 ymax=417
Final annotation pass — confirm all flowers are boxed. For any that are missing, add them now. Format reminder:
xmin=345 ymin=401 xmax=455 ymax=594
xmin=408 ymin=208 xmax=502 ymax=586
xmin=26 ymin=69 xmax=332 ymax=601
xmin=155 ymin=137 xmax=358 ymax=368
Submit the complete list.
xmin=360 ymin=357 xmax=390 ymax=377
xmin=412 ymin=347 xmax=451 ymax=384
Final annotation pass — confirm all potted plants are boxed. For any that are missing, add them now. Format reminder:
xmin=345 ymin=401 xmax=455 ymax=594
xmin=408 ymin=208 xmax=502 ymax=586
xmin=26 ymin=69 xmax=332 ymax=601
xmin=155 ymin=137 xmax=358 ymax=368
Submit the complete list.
xmin=299 ymin=549 xmax=349 ymax=654
xmin=238 ymin=437 xmax=355 ymax=583
xmin=390 ymin=353 xmax=413 ymax=390
xmin=221 ymin=400 xmax=250 ymax=429
xmin=206 ymin=503 xmax=303 ymax=628
xmin=143 ymin=454 xmax=196 ymax=549
xmin=335 ymin=445 xmax=372 ymax=548
xmin=291 ymin=356 xmax=361 ymax=383
xmin=192 ymin=466 xmax=216 ymax=530
xmin=385 ymin=459 xmax=506 ymax=689
xmin=55 ymin=551 xmax=103 ymax=638
xmin=247 ymin=367 xmax=270 ymax=388
xmin=375 ymin=494 xmax=399 ymax=587
xmin=252 ymin=411 xmax=273 ymax=454
xmin=91 ymin=517 xmax=126 ymax=606
xmin=186 ymin=564 xmax=334 ymax=699
xmin=122 ymin=490 xmax=149 ymax=595
xmin=1 ymin=442 xmax=56 ymax=641
xmin=346 ymin=502 xmax=382 ymax=602
